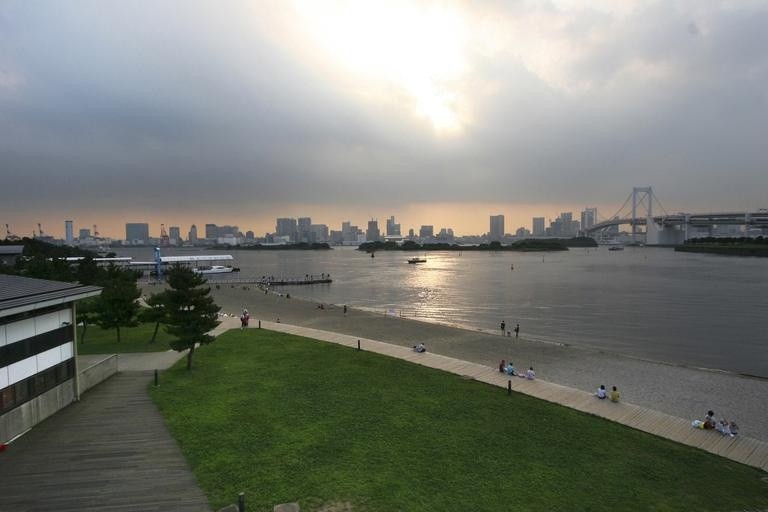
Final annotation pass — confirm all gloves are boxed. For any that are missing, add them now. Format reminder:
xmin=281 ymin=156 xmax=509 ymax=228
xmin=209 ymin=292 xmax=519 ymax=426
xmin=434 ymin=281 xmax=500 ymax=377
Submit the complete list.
xmin=194 ymin=264 xmax=234 ymax=275
xmin=408 ymin=256 xmax=427 ymax=264
xmin=607 ymin=246 xmax=625 ymax=252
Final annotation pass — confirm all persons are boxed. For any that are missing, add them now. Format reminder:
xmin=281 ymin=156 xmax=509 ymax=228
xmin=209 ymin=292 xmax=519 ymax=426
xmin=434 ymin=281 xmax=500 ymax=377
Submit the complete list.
xmin=514 ymin=323 xmax=520 ymax=337
xmin=499 ymin=360 xmax=518 ymax=375
xmin=597 ymin=385 xmax=607 ymax=399
xmin=416 ymin=343 xmax=425 ymax=353
xmin=344 ymin=305 xmax=347 ymax=317
xmin=527 ymin=366 xmax=536 ymax=380
xmin=501 ymin=321 xmax=506 ymax=336
xmin=610 ymin=386 xmax=620 ymax=403
xmin=240 ymin=310 xmax=249 ymax=331
xmin=703 ymin=410 xmax=739 ymax=437
